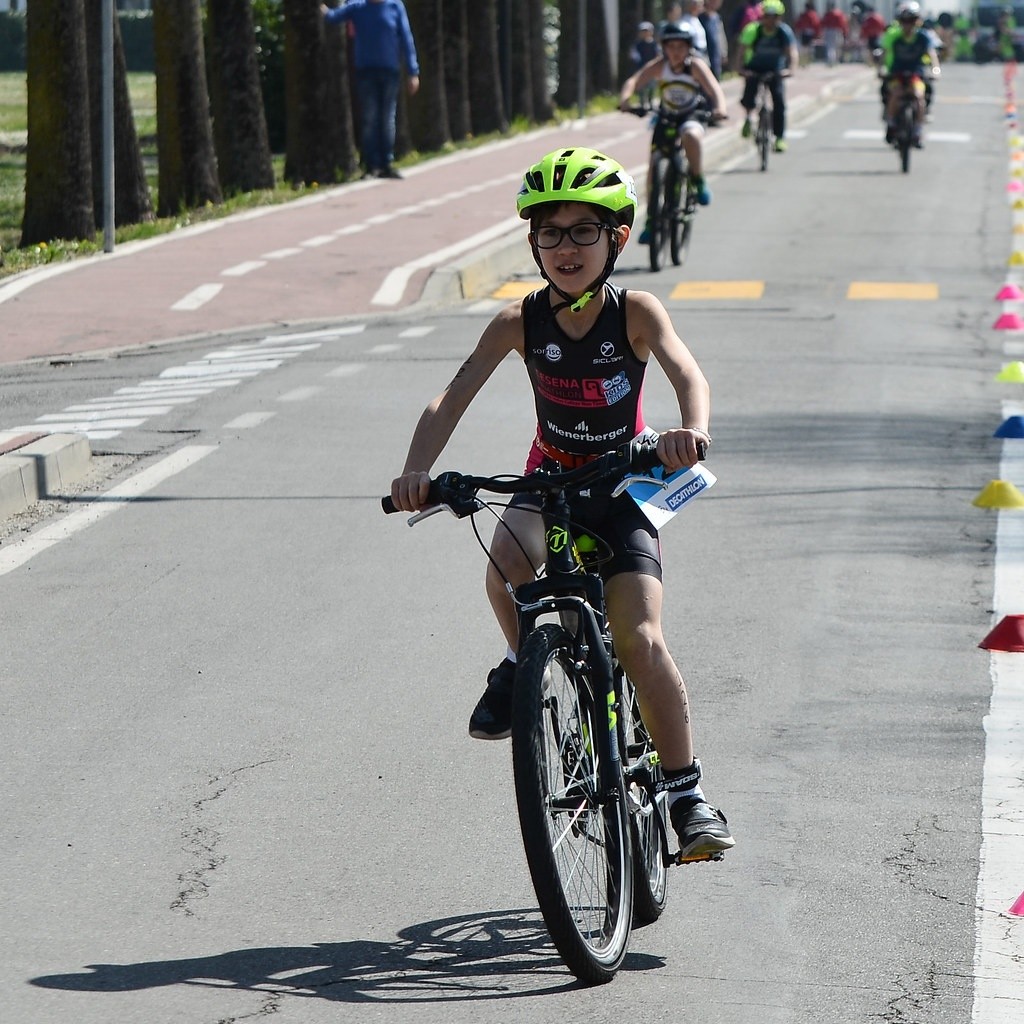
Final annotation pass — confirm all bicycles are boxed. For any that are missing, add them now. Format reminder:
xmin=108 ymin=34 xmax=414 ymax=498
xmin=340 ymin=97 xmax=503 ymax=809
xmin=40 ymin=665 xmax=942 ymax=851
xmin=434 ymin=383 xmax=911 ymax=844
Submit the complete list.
xmin=617 ymin=104 xmax=730 ymax=273
xmin=381 ymin=439 xmax=708 ymax=984
xmin=878 ymin=66 xmax=937 ymax=174
xmin=738 ymin=68 xmax=794 ymax=170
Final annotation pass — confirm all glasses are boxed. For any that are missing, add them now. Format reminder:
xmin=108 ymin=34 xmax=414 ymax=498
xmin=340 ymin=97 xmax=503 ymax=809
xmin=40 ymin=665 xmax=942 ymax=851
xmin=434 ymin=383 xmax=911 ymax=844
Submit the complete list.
xmin=530 ymin=223 xmax=618 ymax=250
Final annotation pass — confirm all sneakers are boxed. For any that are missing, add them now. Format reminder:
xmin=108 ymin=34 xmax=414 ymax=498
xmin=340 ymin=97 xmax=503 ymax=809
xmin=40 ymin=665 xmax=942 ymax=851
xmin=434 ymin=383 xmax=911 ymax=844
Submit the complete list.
xmin=742 ymin=119 xmax=752 ymax=137
xmin=774 ymin=137 xmax=786 ymax=151
xmin=886 ymin=124 xmax=895 ymax=143
xmin=639 ymin=218 xmax=653 ymax=242
xmin=690 ymin=176 xmax=709 ymax=206
xmin=670 ymin=797 xmax=734 ymax=860
xmin=469 ymin=659 xmax=554 ymax=740
xmin=910 ymin=134 xmax=923 ymax=148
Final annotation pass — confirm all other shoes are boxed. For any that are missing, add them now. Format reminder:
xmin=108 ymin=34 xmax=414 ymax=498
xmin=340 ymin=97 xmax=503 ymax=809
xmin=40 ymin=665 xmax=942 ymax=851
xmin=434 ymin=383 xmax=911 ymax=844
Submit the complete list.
xmin=358 ymin=158 xmax=401 ymax=179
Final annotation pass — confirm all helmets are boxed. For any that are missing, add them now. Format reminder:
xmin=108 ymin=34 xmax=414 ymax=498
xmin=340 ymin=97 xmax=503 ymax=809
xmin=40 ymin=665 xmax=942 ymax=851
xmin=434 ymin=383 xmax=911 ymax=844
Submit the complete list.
xmin=895 ymin=1 xmax=921 ymax=20
xmin=660 ymin=20 xmax=696 ymax=60
xmin=760 ymin=0 xmax=785 ymax=15
xmin=517 ymin=147 xmax=638 ymax=311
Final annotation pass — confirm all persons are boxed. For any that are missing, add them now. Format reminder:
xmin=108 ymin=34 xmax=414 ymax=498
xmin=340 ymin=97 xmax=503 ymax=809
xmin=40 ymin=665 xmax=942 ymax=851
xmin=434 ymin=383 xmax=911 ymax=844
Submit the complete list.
xmin=319 ymin=0 xmax=422 ymax=178
xmin=635 ymin=1 xmax=1022 ymax=74
xmin=731 ymin=0 xmax=802 ymax=151
xmin=874 ymin=0 xmax=942 ymax=148
xmin=619 ymin=21 xmax=724 ymax=243
xmin=385 ymin=148 xmax=738 ymax=863
